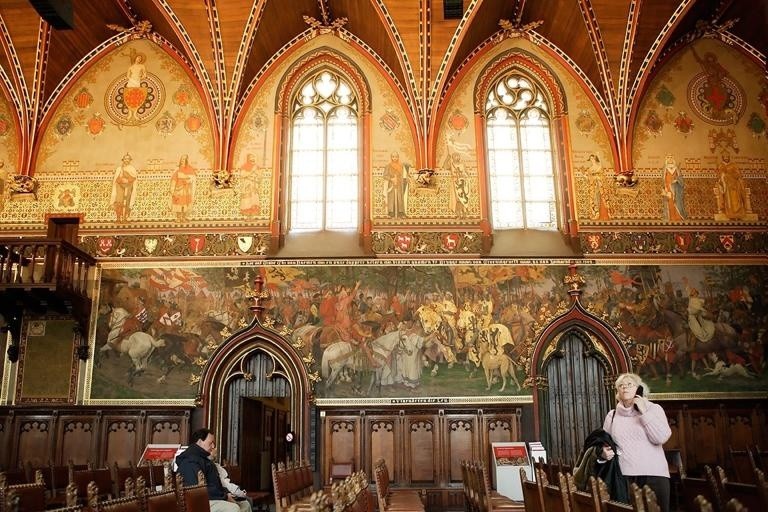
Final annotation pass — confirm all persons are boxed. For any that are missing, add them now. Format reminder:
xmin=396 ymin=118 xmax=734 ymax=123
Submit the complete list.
xmin=585 ymin=154 xmax=610 ymax=221
xmin=166 ymin=153 xmax=196 ymax=222
xmin=660 ymin=152 xmax=686 ymax=221
xmin=172 ymin=428 xmax=252 ymax=512
xmin=125 ymin=54 xmax=147 ymax=121
xmin=690 ymin=45 xmax=729 ymax=112
xmin=600 ymin=372 xmax=674 ymax=510
xmin=442 ymin=152 xmax=471 ymax=219
xmin=716 ymin=149 xmax=745 ymax=219
xmin=382 ymin=150 xmax=412 ymax=219
xmin=205 ymin=441 xmax=255 ymax=508
xmin=238 ymin=153 xmax=262 ymax=221
xmin=108 ymin=152 xmax=138 ymax=220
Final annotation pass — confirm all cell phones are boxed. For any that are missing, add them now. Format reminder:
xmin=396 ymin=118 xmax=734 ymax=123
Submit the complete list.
xmin=633 ymin=385 xmax=643 ymax=412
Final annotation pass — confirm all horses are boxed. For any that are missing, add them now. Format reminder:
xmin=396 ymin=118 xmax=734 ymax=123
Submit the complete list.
xmin=651 ymin=301 xmax=760 ymax=388
xmin=609 ymin=306 xmax=677 ymax=379
xmin=97 ymin=299 xmax=413 ymax=396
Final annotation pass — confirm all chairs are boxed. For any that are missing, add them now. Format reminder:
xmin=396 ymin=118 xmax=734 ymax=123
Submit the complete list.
xmin=0 ymin=459 xmax=270 ymax=512
xmin=269 ymin=456 xmax=427 ymax=512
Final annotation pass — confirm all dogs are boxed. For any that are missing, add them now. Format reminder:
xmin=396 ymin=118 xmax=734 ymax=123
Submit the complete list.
xmin=700 ymin=351 xmax=760 ymax=385
xmin=475 ymin=342 xmax=522 ymax=394
